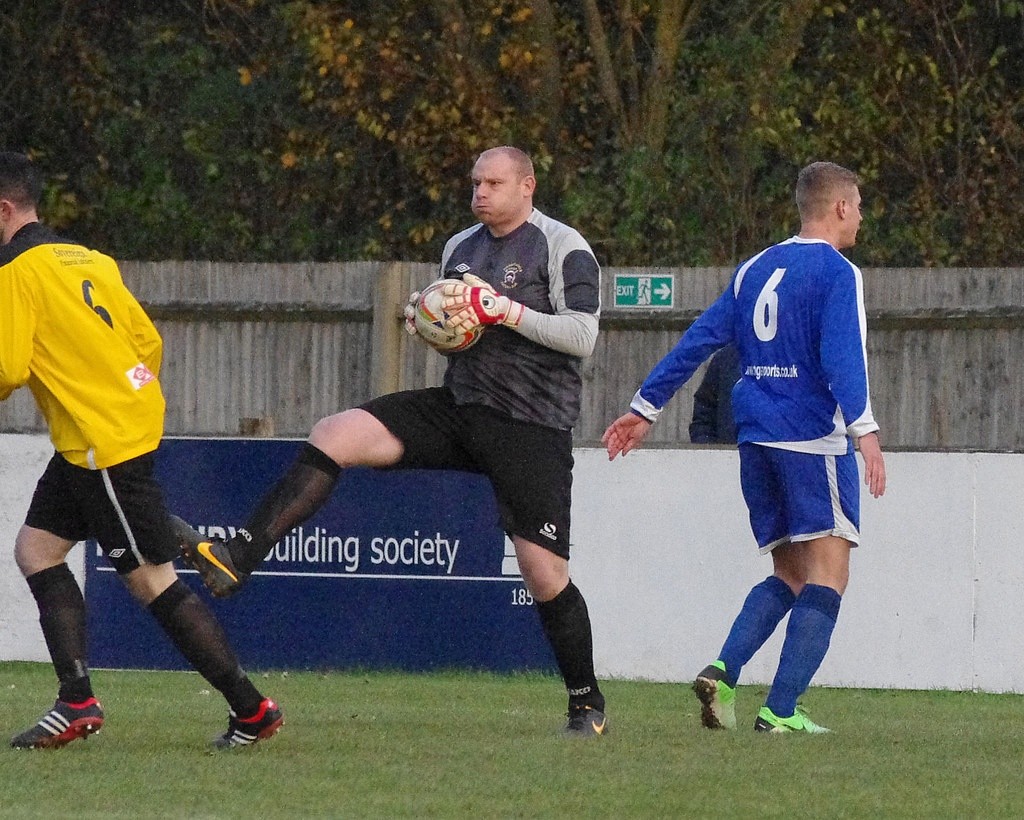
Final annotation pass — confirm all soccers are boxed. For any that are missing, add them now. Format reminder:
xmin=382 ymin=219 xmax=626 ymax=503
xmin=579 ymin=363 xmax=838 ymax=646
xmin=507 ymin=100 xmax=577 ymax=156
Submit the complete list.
xmin=415 ymin=279 xmax=486 ymax=353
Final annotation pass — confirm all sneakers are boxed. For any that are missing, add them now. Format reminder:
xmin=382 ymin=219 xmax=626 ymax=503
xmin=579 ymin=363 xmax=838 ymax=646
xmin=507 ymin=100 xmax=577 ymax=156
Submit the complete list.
xmin=694 ymin=658 xmax=738 ymax=732
xmin=559 ymin=702 xmax=608 ymax=738
xmin=159 ymin=514 xmax=249 ymax=596
xmin=213 ymin=696 xmax=285 ymax=754
xmin=753 ymin=704 xmax=835 ymax=733
xmin=10 ymin=698 xmax=106 ymax=749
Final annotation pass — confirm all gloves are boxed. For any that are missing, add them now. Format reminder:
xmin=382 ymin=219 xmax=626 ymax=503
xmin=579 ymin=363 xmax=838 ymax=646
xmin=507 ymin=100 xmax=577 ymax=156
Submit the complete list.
xmin=401 ymin=291 xmax=420 ymax=334
xmin=440 ymin=270 xmax=527 ymax=336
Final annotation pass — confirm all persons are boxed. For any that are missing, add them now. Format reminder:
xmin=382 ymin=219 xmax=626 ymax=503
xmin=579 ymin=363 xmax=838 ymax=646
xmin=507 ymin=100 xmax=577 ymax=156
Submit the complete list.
xmin=0 ymin=151 xmax=285 ymax=751
xmin=168 ymin=146 xmax=607 ymax=739
xmin=600 ymin=162 xmax=886 ymax=733
